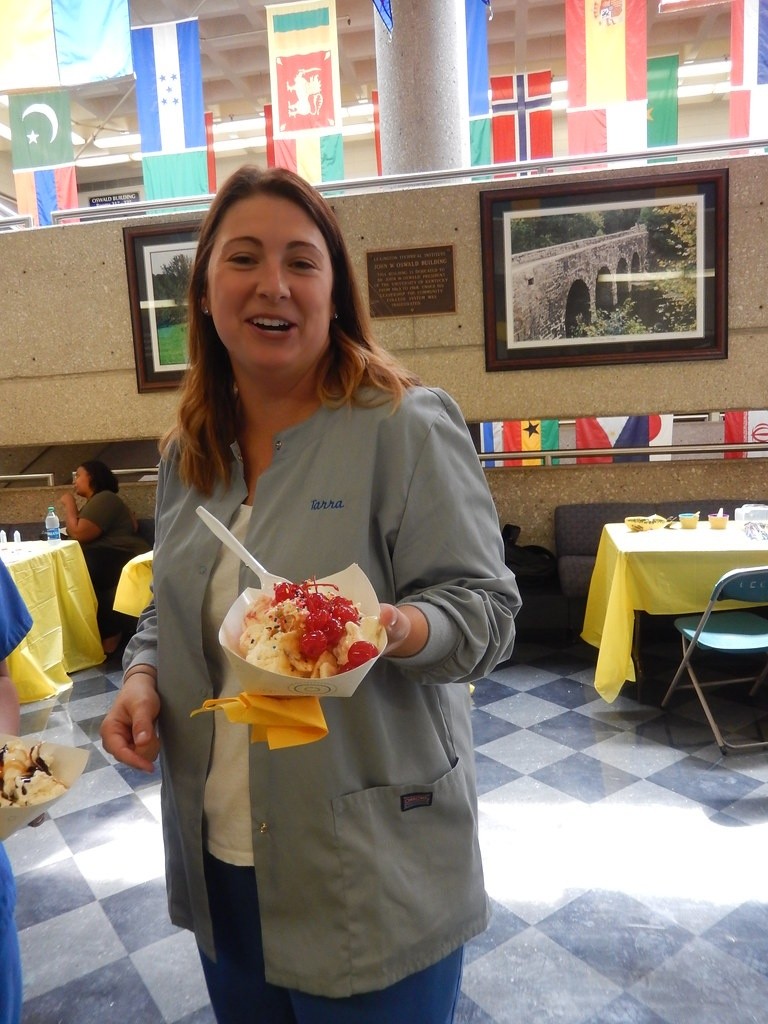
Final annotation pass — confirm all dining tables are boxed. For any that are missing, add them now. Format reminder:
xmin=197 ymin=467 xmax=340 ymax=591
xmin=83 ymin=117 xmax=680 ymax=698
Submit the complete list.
xmin=579 ymin=520 xmax=768 ymax=705
xmin=0 ymin=540 xmax=108 ymax=704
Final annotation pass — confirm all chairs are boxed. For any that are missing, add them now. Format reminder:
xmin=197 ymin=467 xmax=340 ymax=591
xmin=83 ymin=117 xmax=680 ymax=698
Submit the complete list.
xmin=659 ymin=565 xmax=768 ymax=756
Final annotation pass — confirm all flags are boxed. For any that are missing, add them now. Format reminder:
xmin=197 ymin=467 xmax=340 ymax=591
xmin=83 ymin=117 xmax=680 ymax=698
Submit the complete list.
xmin=480 ymin=409 xmax=768 ymax=468
xmin=0 ymin=0 xmax=768 ymax=228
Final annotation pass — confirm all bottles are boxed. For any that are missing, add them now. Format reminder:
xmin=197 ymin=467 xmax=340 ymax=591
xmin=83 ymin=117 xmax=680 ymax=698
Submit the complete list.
xmin=14 ymin=530 xmax=20 ymax=549
xmin=0 ymin=530 xmax=7 ymax=550
xmin=45 ymin=506 xmax=61 ymax=545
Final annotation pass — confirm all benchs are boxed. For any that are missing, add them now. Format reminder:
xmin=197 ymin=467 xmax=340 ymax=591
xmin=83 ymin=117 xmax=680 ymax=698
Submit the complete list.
xmin=554 ymin=499 xmax=768 ymax=644
xmin=0 ymin=518 xmax=154 ymax=548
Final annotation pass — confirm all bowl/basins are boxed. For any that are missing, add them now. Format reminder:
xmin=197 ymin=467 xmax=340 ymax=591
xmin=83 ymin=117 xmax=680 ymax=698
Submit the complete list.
xmin=679 ymin=514 xmax=699 ymax=529
xmin=709 ymin=514 xmax=729 ymax=529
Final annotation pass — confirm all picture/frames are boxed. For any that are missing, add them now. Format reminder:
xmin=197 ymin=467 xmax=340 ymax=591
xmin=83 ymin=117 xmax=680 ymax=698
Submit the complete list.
xmin=478 ymin=168 xmax=729 ymax=372
xmin=122 ymin=219 xmax=204 ymax=394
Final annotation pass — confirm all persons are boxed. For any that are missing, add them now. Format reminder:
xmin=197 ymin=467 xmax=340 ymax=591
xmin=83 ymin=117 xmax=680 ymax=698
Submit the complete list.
xmin=60 ymin=459 xmax=145 ymax=664
xmin=99 ymin=164 xmax=524 ymax=1024
xmin=0 ymin=554 xmax=45 ymax=1024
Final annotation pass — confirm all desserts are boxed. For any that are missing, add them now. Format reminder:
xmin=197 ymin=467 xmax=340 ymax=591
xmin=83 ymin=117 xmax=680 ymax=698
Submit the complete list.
xmin=237 ymin=581 xmax=383 ymax=680
xmin=0 ymin=736 xmax=72 ymax=808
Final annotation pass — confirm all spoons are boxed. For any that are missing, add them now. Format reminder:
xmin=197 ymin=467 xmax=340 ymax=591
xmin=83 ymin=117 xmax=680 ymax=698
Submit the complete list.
xmin=196 ymin=506 xmax=292 ymax=598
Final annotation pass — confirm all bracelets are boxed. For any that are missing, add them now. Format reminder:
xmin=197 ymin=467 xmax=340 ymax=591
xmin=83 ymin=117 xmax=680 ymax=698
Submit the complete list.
xmin=123 ymin=664 xmax=157 ymax=683
